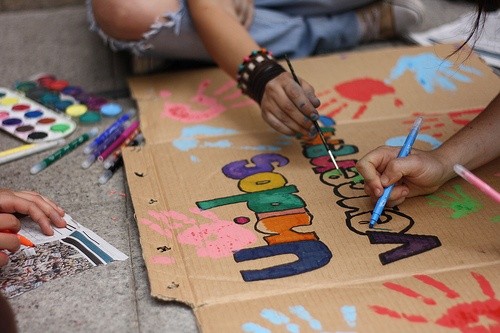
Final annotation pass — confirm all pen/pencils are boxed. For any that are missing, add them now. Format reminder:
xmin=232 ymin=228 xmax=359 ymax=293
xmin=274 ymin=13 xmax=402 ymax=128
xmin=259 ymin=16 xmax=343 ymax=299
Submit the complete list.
xmin=31 ymin=127 xmax=97 ymax=175
xmin=0 ymin=139 xmax=66 ymax=165
xmin=16 ymin=233 xmax=35 ymax=247
xmin=453 ymin=163 xmax=500 ymax=205
xmin=369 ymin=117 xmax=422 ymax=228
xmin=82 ymin=109 xmax=143 ymax=183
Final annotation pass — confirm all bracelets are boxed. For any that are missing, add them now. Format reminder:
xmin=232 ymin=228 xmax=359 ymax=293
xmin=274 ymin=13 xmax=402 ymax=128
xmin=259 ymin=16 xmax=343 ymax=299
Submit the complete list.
xmin=236 ymin=48 xmax=287 ymax=107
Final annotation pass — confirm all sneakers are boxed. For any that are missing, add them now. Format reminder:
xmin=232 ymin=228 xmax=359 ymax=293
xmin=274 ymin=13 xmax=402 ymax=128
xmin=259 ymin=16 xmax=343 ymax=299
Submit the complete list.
xmin=355 ymin=0 xmax=426 ymax=45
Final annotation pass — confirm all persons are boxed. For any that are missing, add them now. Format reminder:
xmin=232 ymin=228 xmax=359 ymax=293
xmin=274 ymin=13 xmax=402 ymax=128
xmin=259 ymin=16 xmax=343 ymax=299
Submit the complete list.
xmin=354 ymin=0 xmax=500 ymax=210
xmin=85 ymin=0 xmax=426 ymax=141
xmin=0 ymin=187 xmax=67 ymax=268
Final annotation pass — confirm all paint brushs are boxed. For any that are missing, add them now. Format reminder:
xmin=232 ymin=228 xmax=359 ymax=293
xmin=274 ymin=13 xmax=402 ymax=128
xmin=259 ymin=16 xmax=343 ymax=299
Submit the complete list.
xmin=283 ymin=53 xmax=343 ymax=177
